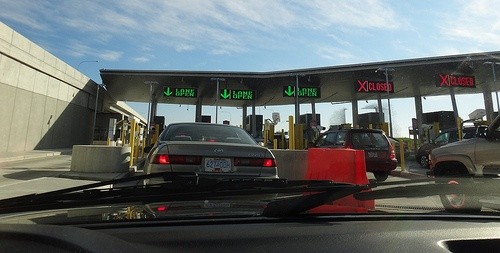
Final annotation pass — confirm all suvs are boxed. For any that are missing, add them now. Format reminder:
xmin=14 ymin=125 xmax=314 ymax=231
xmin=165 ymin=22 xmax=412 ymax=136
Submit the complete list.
xmin=430 ymin=112 xmax=500 ymax=211
xmin=309 ymin=128 xmax=398 ymax=181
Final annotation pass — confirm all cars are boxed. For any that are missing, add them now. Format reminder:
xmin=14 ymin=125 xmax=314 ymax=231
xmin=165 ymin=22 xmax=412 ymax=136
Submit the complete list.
xmin=387 ymin=137 xmax=409 ymax=162
xmin=141 ymin=121 xmax=286 ymax=187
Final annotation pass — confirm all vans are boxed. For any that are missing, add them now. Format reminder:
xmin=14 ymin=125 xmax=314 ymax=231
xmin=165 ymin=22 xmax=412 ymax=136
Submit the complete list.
xmin=415 ymin=126 xmax=487 ymax=167
